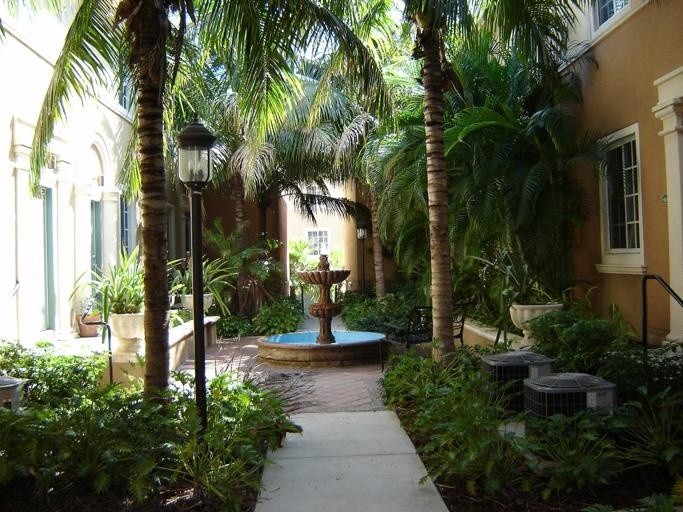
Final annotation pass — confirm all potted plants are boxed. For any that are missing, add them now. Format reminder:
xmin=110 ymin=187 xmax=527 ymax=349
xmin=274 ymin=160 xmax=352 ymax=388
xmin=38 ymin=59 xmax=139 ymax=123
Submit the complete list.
xmin=69 ymin=276 xmax=102 ymax=337
xmin=463 ymin=251 xmax=565 ymax=350
xmin=168 ymin=252 xmax=240 ymax=321
xmin=68 ymin=252 xmax=145 ymax=354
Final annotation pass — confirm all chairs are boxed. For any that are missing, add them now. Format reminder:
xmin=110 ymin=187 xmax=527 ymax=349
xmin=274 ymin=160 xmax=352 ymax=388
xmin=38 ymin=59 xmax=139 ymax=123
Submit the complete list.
xmin=385 ymin=303 xmax=469 ymax=356
